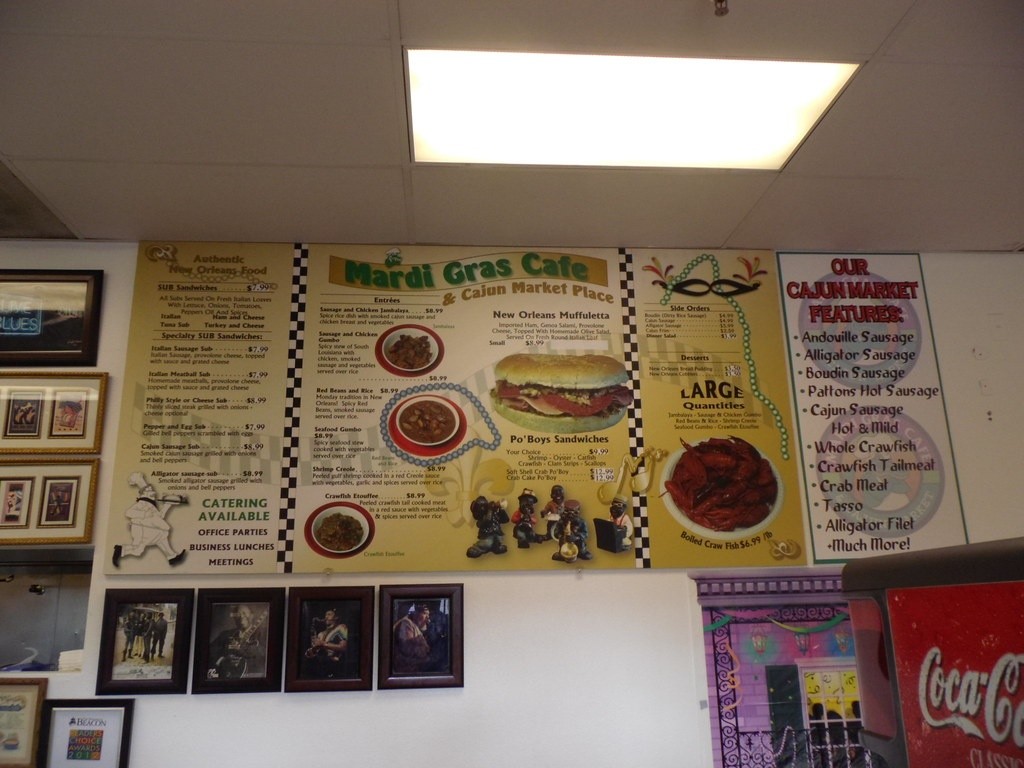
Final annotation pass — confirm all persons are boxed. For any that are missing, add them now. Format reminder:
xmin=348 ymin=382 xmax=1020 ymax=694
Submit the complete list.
xmin=121 ymin=601 xmax=442 ymax=677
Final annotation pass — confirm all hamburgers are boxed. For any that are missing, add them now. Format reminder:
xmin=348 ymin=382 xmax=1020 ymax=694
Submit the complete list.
xmin=489 ymin=353 xmax=634 ymax=434
